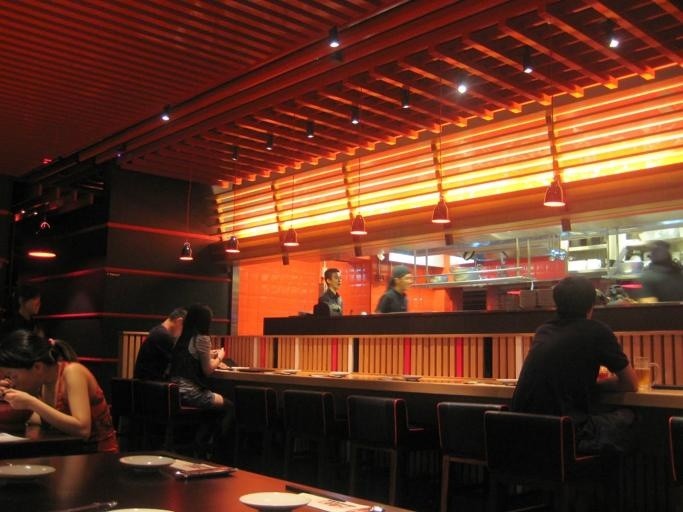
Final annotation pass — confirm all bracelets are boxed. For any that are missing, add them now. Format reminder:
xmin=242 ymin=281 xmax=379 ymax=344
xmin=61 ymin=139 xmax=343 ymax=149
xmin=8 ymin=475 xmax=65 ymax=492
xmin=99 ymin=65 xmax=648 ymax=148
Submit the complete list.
xmin=216 ymin=354 xmax=222 ymax=363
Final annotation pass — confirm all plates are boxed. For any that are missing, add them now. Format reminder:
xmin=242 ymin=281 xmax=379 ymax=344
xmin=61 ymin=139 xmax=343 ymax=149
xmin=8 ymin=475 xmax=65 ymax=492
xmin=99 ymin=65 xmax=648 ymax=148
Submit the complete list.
xmin=400 ymin=375 xmax=423 ymax=381
xmin=0 ymin=432 xmax=28 ymax=442
xmin=237 ymin=492 xmax=309 ymax=512
xmin=281 ymin=370 xmax=299 ymax=374
xmin=330 ymin=372 xmax=350 ymax=378
xmin=105 ymin=508 xmax=172 ymax=512
xmin=118 ymin=454 xmax=175 ymax=473
xmin=495 ymin=378 xmax=518 ymax=385
xmin=517 ymin=288 xmax=556 ymax=310
xmin=0 ymin=464 xmax=57 ymax=480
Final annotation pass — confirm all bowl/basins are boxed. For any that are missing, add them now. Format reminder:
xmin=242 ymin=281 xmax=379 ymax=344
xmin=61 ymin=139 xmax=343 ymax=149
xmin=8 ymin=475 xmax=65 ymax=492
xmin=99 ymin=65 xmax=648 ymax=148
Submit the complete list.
xmin=618 ymin=262 xmax=643 ymax=275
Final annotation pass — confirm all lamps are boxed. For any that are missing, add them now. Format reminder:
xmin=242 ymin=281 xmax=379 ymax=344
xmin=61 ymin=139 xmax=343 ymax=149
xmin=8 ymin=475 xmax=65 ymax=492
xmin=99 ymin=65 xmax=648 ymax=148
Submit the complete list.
xmin=541 ymin=52 xmax=566 ymax=208
xmin=177 ymin=147 xmax=195 ymax=263
xmin=27 ymin=209 xmax=57 ymax=262
xmin=348 ymin=100 xmax=368 ymax=236
xmin=281 ymin=107 xmax=302 ymax=248
xmin=225 ymin=140 xmax=241 ymax=255
xmin=428 ymin=60 xmax=452 ymax=224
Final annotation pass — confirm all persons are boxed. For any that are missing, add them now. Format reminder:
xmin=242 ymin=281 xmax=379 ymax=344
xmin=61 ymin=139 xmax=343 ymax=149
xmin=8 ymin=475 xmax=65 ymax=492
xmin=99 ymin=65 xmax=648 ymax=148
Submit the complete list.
xmin=0 ymin=326 xmax=120 ymax=454
xmin=1 ymin=285 xmax=46 ymax=344
xmin=318 ymin=268 xmax=343 ymax=316
xmin=164 ymin=301 xmax=238 ymax=454
xmin=507 ymin=276 xmax=640 ymax=468
xmin=637 ymin=237 xmax=683 ymax=302
xmin=372 ymin=265 xmax=412 ymax=313
xmin=134 ymin=309 xmax=189 ymax=376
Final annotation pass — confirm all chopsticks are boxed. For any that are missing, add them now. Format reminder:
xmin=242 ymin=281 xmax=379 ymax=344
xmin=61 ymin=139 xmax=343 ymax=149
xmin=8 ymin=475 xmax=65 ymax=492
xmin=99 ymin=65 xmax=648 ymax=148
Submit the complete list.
xmin=283 ymin=483 xmax=347 ymax=502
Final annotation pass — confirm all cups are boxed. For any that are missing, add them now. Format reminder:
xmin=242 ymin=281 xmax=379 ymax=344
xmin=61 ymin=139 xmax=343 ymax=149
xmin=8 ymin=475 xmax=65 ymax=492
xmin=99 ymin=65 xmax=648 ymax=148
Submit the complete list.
xmin=634 ymin=355 xmax=659 ymax=391
xmin=605 ymin=267 xmax=618 ymax=276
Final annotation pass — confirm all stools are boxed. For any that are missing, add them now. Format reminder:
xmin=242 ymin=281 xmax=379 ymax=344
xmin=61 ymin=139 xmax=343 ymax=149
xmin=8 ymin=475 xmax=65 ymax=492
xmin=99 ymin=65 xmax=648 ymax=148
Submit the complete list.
xmin=665 ymin=412 xmax=681 ymax=502
xmin=131 ymin=377 xmax=219 ymax=462
xmin=231 ymin=380 xmax=286 ymax=475
xmin=277 ymin=383 xmax=345 ymax=479
xmin=482 ymin=409 xmax=599 ymax=512
xmin=343 ymin=390 xmax=429 ymax=506
xmin=112 ymin=376 xmax=138 ymax=443
xmin=432 ymin=399 xmax=509 ymax=508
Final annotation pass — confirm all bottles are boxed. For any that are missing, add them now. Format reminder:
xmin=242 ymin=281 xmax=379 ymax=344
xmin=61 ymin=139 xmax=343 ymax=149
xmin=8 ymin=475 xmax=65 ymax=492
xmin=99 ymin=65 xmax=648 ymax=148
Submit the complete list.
xmin=320 ymin=260 xmax=329 ymax=294
